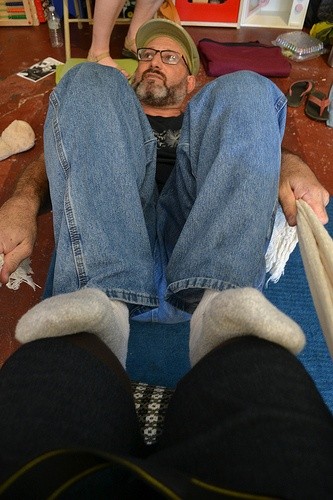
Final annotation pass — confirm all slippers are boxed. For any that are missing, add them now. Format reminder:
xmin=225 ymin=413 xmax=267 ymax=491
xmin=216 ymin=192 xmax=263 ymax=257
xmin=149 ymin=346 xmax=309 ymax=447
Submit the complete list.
xmin=305 ymin=90 xmax=330 ymax=121
xmin=284 ymin=80 xmax=315 ymax=107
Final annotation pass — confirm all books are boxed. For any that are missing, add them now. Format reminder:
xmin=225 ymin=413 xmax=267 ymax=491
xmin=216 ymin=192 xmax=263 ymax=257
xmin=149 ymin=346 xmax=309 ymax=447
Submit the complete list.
xmin=16 ymin=57 xmax=65 ymax=83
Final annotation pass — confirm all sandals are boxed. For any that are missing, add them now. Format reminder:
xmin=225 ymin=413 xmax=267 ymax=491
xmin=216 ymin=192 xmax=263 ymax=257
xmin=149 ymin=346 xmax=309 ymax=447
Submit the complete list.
xmin=88 ymin=55 xmax=131 ymax=80
xmin=122 ymin=38 xmax=139 ymax=60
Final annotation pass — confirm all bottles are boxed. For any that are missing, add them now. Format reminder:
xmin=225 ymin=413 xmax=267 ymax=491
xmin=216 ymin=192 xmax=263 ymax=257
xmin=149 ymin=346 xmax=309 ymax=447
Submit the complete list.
xmin=48 ymin=6 xmax=63 ymax=48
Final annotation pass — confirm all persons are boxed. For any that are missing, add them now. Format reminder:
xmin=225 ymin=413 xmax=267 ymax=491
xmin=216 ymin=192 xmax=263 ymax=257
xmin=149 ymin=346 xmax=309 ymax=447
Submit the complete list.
xmin=0 ymin=332 xmax=333 ymax=500
xmin=86 ymin=0 xmax=165 ymax=80
xmin=0 ymin=19 xmax=329 ymax=370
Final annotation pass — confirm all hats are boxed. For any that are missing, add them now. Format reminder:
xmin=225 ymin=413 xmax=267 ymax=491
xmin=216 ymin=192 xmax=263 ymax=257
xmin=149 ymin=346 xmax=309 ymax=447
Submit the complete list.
xmin=135 ymin=19 xmax=200 ymax=77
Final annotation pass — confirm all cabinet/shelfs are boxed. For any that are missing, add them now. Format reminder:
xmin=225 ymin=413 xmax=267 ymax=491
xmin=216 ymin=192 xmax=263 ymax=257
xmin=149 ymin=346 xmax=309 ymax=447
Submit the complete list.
xmin=0 ymin=0 xmax=333 ymax=30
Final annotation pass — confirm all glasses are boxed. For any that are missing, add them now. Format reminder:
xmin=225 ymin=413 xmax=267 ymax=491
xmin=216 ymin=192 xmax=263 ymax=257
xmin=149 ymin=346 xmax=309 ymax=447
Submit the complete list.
xmin=137 ymin=47 xmax=194 ymax=76
